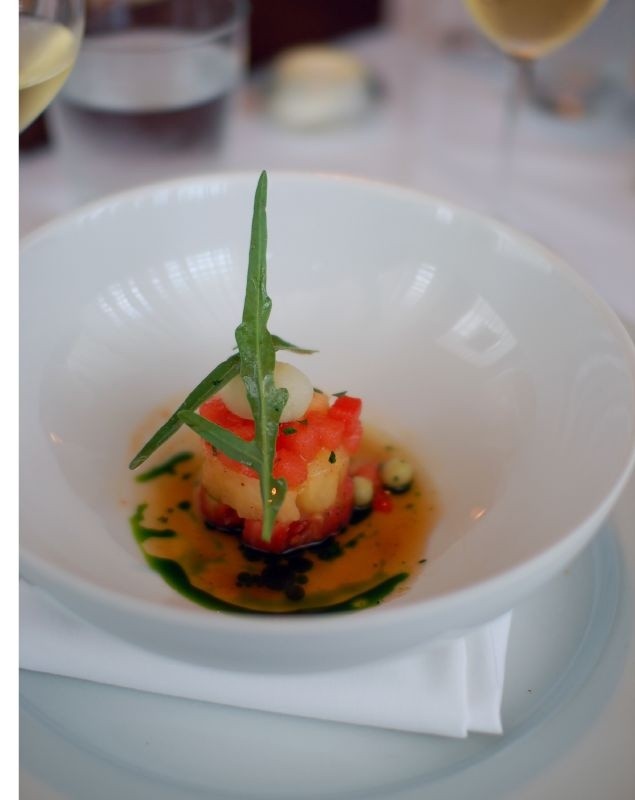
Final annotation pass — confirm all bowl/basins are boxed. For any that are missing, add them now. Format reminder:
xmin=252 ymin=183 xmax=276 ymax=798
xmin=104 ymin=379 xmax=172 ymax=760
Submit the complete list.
xmin=20 ymin=169 xmax=635 ymax=678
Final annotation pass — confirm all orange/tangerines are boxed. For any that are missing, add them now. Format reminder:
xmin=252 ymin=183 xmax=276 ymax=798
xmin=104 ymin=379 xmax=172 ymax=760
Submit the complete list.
xmin=20 ymin=34 xmax=78 ymax=132
xmin=465 ymin=0 xmax=609 ymax=62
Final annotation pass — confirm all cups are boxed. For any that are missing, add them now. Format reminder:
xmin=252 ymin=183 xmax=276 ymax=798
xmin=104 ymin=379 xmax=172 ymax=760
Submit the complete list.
xmin=18 ymin=0 xmax=85 ymax=138
xmin=41 ymin=0 xmax=247 ymax=191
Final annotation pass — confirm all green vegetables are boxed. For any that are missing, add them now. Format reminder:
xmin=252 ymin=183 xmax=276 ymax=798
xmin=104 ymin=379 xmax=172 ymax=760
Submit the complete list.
xmin=127 ymin=171 xmax=322 ymax=545
